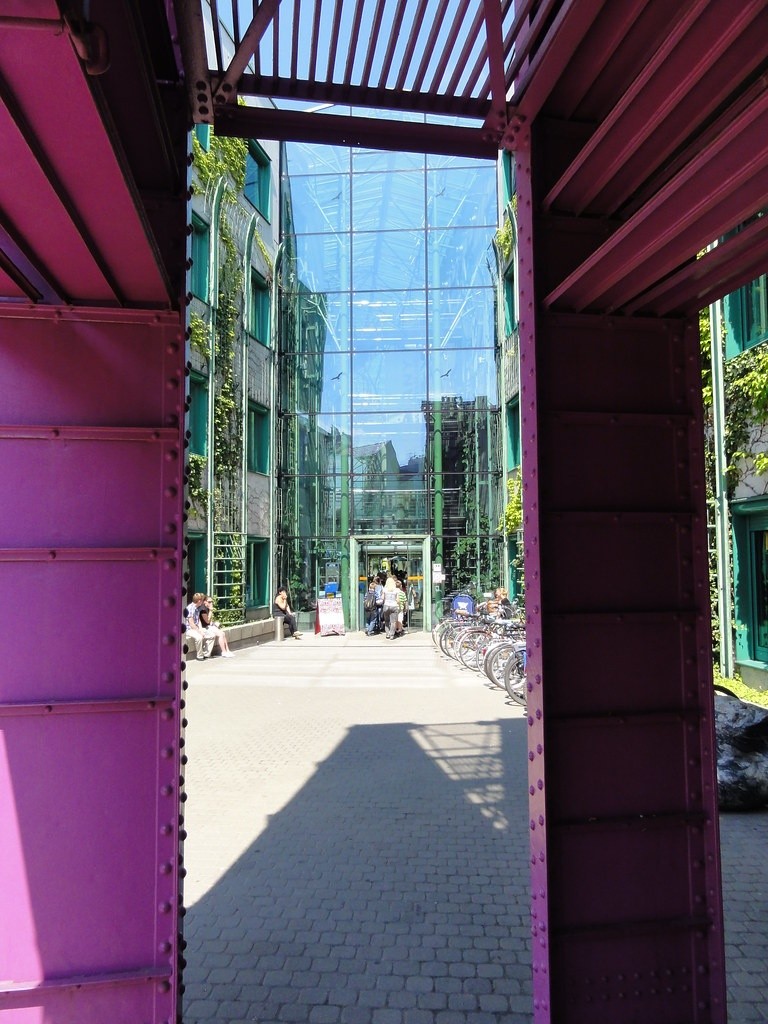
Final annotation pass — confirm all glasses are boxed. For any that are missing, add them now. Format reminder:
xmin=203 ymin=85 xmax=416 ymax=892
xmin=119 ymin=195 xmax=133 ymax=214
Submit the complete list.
xmin=206 ymin=601 xmax=213 ymax=603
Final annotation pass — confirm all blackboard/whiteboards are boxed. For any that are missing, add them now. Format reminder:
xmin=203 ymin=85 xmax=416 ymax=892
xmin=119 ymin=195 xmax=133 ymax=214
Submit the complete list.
xmin=317 ymin=598 xmax=345 ymax=636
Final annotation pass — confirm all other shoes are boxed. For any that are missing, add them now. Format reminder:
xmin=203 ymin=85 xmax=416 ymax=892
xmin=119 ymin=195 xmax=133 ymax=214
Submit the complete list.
xmin=292 ymin=631 xmax=303 ymax=636
xmin=222 ymin=651 xmax=235 ymax=657
xmin=365 ymin=629 xmax=374 ymax=636
xmin=386 ymin=634 xmax=394 ymax=640
xmin=197 ymin=656 xmax=213 ymax=662
xmin=295 ymin=636 xmax=301 ymax=640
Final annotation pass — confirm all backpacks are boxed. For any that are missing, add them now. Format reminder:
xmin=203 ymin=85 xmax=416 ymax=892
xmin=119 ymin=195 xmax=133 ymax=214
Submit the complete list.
xmin=364 ymin=592 xmax=376 ymax=611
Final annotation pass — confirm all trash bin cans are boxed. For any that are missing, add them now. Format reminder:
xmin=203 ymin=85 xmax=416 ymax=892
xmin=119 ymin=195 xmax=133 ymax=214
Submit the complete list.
xmin=324 ymin=581 xmax=338 ymax=598
xmin=452 ymin=594 xmax=475 ymax=618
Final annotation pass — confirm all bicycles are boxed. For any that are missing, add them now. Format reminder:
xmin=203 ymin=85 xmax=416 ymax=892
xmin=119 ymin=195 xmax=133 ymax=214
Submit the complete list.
xmin=433 ymin=593 xmax=525 ymax=707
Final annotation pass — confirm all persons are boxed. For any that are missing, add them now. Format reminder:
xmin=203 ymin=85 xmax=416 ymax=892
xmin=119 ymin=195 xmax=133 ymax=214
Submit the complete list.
xmin=272 ymin=587 xmax=303 ymax=637
xmin=494 ymin=589 xmax=513 ymax=620
xmin=184 ymin=592 xmax=236 ymax=661
xmin=364 ymin=569 xmax=417 ymax=640
